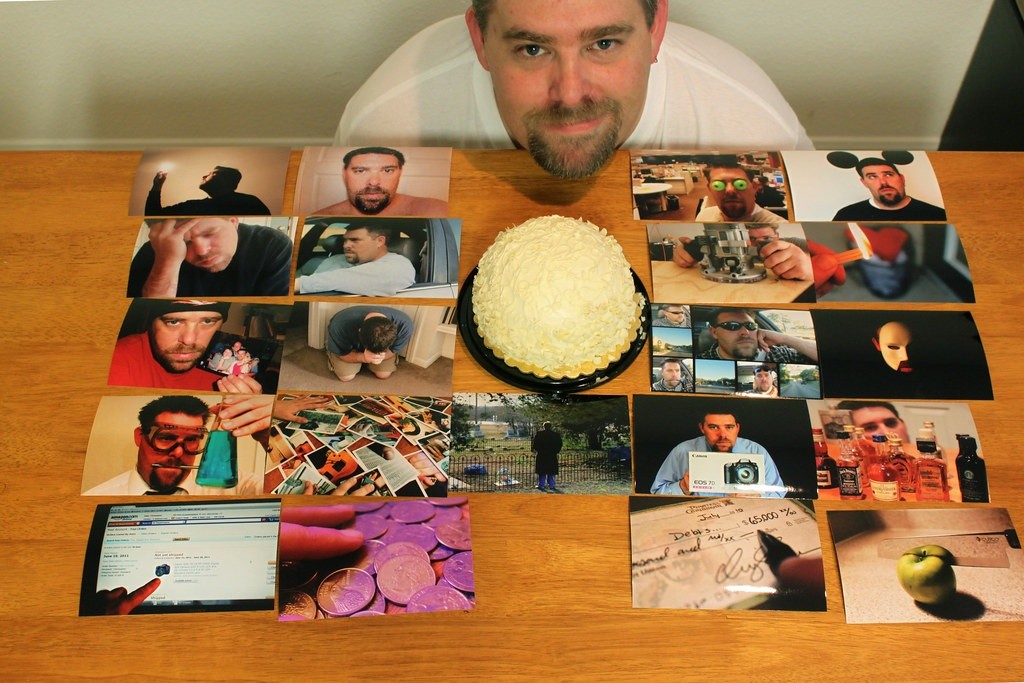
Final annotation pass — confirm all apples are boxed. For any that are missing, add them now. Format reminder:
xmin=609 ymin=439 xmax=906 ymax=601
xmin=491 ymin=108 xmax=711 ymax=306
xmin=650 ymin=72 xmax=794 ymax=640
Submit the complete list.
xmin=897 ymin=544 xmax=957 ymax=604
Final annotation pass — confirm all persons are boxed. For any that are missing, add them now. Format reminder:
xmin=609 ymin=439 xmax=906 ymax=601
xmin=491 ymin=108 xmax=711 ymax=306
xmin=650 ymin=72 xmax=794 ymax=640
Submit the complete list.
xmin=534 ymin=419 xmax=561 ymax=489
xmin=82 ymin=396 xmax=273 ymax=497
xmin=265 ymin=388 xmax=454 ymax=498
xmin=695 ymin=160 xmax=793 ymax=223
xmin=84 ymin=578 xmax=162 ymax=616
xmin=652 ymin=303 xmax=818 ymax=398
xmin=333 ymin=0 xmax=816 ymax=180
xmin=869 ymin=319 xmax=933 ymax=395
xmin=143 ymin=165 xmax=272 ymax=216
xmin=778 ymin=557 xmax=825 ymax=589
xmin=835 ymin=399 xmax=910 ymax=446
xmin=278 ymin=504 xmax=366 ymax=563
xmin=312 ymin=146 xmax=450 ymax=217
xmin=323 ymin=305 xmax=414 ymax=380
xmin=109 ymin=300 xmax=264 ymax=395
xmin=294 ymin=219 xmax=418 ymax=298
xmin=831 ymin=158 xmax=948 ymax=222
xmin=126 ymin=214 xmax=292 ymax=297
xmin=207 ymin=341 xmax=259 ymax=376
xmin=675 ymin=224 xmax=814 ymax=285
xmin=648 ymin=410 xmax=788 ymax=498
xmin=808 ymin=238 xmax=846 ymax=296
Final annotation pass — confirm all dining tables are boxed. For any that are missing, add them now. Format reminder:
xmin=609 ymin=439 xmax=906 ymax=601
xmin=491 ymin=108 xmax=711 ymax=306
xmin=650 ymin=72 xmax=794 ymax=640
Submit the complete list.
xmin=3 ymin=146 xmax=1024 ymax=681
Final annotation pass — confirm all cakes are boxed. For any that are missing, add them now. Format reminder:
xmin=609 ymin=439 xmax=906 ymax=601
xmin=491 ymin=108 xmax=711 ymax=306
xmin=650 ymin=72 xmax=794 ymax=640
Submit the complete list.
xmin=470 ymin=213 xmax=646 ymax=378
